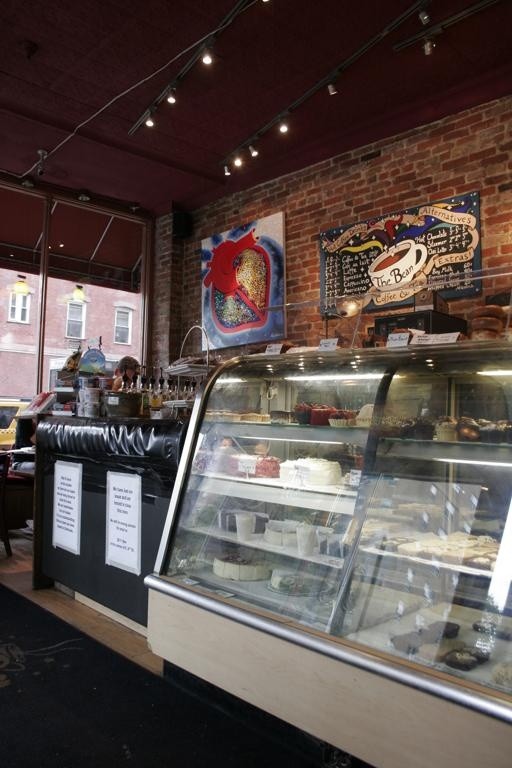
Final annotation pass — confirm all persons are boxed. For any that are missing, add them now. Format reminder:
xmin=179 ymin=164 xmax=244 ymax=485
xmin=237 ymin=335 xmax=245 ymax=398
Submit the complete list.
xmin=109 ymin=356 xmax=141 ymax=390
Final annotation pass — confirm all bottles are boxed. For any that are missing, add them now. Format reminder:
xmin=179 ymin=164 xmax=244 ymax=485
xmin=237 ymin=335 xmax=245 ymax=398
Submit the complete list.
xmin=121 ymin=375 xmax=196 ymax=417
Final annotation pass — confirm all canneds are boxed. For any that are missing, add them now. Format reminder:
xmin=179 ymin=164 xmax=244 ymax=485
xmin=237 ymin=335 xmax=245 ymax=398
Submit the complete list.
xmin=75 ymin=376 xmax=115 ymax=417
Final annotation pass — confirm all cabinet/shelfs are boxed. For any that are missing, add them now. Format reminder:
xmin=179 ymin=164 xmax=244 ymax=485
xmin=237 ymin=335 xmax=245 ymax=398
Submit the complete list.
xmin=142 ymin=339 xmax=512 ymax=768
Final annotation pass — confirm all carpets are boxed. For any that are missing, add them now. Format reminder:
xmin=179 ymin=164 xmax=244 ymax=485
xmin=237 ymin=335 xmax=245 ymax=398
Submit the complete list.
xmin=0 ymin=580 xmax=292 ymax=768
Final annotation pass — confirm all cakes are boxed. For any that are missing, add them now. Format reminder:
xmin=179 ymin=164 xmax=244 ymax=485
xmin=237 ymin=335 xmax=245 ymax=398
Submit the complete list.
xmin=203 ymin=400 xmax=511 ymax=671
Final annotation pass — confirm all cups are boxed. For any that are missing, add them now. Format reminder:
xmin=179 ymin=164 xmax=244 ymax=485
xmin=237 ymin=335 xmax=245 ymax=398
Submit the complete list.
xmin=294 ymin=526 xmax=315 ymax=557
xmin=369 ymin=238 xmax=427 ymax=292
xmin=234 ymin=515 xmax=255 ymax=539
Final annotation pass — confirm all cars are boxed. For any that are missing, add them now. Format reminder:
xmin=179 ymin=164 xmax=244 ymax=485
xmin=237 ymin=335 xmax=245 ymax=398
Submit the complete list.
xmin=0 ymin=396 xmax=33 ymax=452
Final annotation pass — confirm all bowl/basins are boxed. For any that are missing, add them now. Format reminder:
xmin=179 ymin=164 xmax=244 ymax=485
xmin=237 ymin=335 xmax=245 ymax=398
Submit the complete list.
xmin=104 ymin=391 xmax=140 ymax=418
